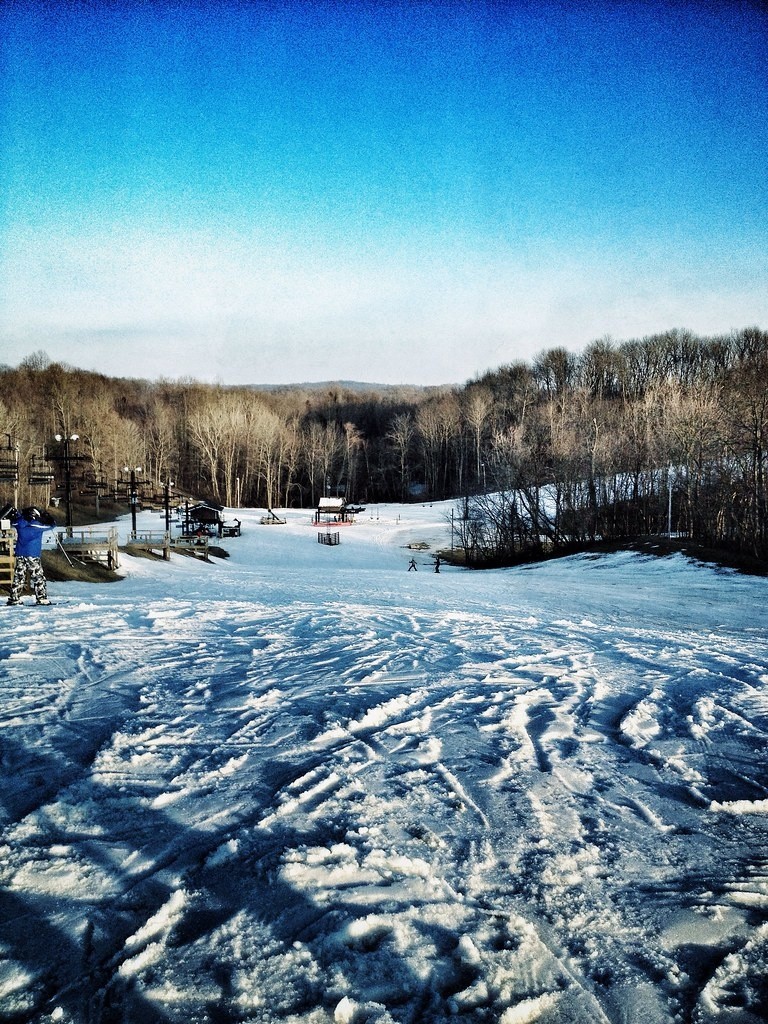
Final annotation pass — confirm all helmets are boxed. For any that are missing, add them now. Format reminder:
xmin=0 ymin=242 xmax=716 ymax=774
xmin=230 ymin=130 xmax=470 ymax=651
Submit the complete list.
xmin=22 ymin=506 xmax=41 ymax=520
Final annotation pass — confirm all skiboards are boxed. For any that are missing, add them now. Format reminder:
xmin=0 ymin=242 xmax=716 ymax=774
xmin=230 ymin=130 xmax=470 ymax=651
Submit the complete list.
xmin=0 ymin=600 xmax=70 ymax=606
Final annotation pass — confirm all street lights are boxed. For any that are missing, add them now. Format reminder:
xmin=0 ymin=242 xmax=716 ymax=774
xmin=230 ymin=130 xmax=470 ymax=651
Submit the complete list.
xmin=236 ymin=478 xmax=239 ymax=508
xmin=124 ymin=466 xmax=142 ymax=538
xmin=54 ymin=434 xmax=80 ymax=537
xmin=481 ymin=464 xmax=486 ymax=496
xmin=161 ymin=482 xmax=174 ymax=530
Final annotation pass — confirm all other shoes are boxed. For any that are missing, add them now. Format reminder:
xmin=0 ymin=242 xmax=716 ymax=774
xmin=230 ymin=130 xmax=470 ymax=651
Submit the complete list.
xmin=36 ymin=598 xmax=51 ymax=605
xmin=6 ymin=599 xmax=24 ymax=605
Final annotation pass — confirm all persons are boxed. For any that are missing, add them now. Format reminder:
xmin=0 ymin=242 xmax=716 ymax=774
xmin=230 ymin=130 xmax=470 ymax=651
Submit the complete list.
xmin=1 ymin=503 xmax=56 ymax=605
xmin=435 ymin=557 xmax=440 ymax=573
xmin=408 ymin=559 xmax=418 ymax=571
